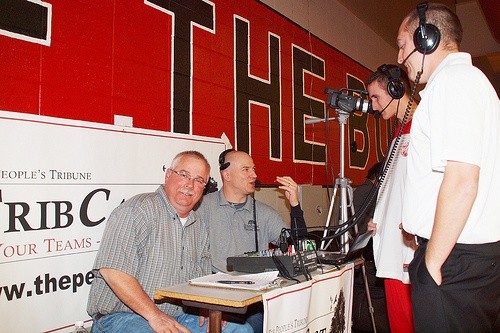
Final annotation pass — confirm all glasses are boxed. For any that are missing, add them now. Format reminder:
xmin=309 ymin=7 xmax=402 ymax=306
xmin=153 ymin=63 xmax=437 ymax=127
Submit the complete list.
xmin=170 ymin=169 xmax=207 ymax=188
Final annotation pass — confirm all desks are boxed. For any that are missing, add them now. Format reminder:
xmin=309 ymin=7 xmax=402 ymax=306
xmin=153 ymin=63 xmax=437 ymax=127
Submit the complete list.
xmin=154 ymin=259 xmax=364 ymax=333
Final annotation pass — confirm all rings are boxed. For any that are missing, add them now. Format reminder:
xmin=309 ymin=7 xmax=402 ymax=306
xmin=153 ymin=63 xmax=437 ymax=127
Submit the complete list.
xmin=288 ymin=184 xmax=291 ymax=187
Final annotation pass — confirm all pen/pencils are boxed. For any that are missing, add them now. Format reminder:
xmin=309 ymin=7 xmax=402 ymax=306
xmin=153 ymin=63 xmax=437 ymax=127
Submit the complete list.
xmin=217 ymin=281 xmax=255 ymax=284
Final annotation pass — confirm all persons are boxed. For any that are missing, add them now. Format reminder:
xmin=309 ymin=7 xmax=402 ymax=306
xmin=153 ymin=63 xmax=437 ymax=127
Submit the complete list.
xmin=397 ymin=7 xmax=500 ymax=333
xmin=197 ymin=149 xmax=308 ymax=333
xmin=348 ymin=162 xmax=385 ymax=239
xmin=367 ymin=66 xmax=419 ymax=333
xmin=86 ymin=151 xmax=255 ymax=333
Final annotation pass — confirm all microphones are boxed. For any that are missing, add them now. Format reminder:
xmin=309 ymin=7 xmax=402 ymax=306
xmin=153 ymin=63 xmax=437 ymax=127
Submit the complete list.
xmin=374 ymin=98 xmax=395 ymax=118
xmin=395 ymin=48 xmax=418 ymax=71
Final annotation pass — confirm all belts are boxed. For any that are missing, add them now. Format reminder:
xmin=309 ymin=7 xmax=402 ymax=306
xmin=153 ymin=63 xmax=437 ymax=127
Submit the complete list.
xmin=414 ymin=235 xmax=428 ymax=246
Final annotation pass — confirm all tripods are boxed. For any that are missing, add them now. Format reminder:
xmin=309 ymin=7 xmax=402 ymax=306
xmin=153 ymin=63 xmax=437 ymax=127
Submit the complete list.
xmin=319 ymin=111 xmax=376 ymax=333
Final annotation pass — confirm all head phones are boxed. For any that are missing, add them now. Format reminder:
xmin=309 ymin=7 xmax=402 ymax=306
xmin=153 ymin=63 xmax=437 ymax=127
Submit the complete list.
xmin=412 ymin=2 xmax=440 ymax=54
xmin=377 ymin=64 xmax=405 ymax=99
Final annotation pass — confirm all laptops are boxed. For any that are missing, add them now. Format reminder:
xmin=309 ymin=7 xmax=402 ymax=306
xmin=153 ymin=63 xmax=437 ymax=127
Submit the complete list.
xmin=319 ymin=229 xmax=374 ymax=264
